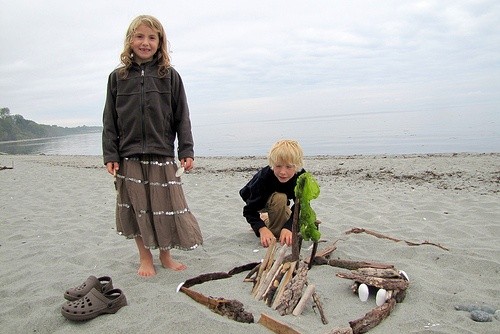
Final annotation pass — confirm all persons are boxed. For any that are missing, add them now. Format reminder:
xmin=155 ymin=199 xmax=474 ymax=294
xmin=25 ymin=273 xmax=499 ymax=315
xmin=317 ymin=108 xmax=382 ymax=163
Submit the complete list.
xmin=102 ymin=15 xmax=202 ymax=278
xmin=241 ymin=139 xmax=310 ymax=247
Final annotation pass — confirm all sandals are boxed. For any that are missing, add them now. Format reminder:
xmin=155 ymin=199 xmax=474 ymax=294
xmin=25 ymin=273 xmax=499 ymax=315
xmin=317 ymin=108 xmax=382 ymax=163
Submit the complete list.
xmin=63 ymin=275 xmax=113 ymax=301
xmin=61 ymin=288 xmax=127 ymax=321
